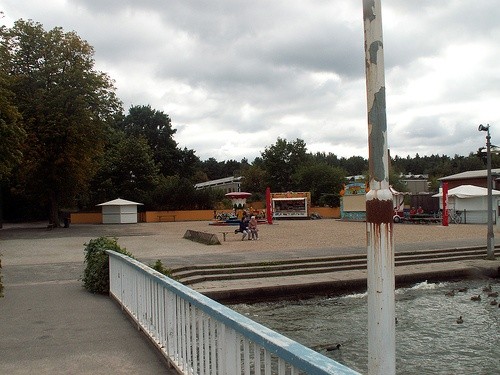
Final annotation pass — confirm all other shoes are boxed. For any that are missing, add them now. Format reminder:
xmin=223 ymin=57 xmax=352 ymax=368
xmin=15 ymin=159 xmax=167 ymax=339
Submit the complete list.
xmin=252 ymin=239 xmax=254 ymax=240
xmin=248 ymin=239 xmax=252 ymax=240
xmin=256 ymin=238 xmax=259 ymax=241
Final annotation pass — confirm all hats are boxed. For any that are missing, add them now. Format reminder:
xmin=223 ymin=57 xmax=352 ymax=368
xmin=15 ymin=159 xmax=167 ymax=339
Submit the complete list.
xmin=251 ymin=216 xmax=255 ymax=218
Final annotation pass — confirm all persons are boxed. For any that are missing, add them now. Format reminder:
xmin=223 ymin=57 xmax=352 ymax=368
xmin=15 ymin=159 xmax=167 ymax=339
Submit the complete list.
xmin=241 ymin=209 xmax=265 ymax=218
xmin=409 ymin=207 xmax=416 ymax=223
xmin=240 ymin=217 xmax=252 ymax=241
xmin=249 ymin=216 xmax=259 ymax=240
xmin=416 ymin=206 xmax=423 ymax=224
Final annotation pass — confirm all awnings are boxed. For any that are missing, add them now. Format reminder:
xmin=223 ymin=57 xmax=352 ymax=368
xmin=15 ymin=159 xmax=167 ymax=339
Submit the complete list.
xmin=271 ymin=198 xmax=304 ymax=200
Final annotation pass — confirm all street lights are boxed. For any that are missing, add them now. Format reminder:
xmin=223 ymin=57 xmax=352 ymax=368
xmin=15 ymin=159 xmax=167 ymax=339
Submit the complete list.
xmin=478 ymin=124 xmax=496 ymax=259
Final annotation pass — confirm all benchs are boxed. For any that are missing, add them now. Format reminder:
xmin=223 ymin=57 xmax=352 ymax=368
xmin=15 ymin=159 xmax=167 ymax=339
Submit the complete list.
xmin=47 ymin=223 xmax=54 ymax=231
xmin=156 ymin=214 xmax=178 ymax=222
xmin=218 ymin=231 xmax=242 ymax=242
